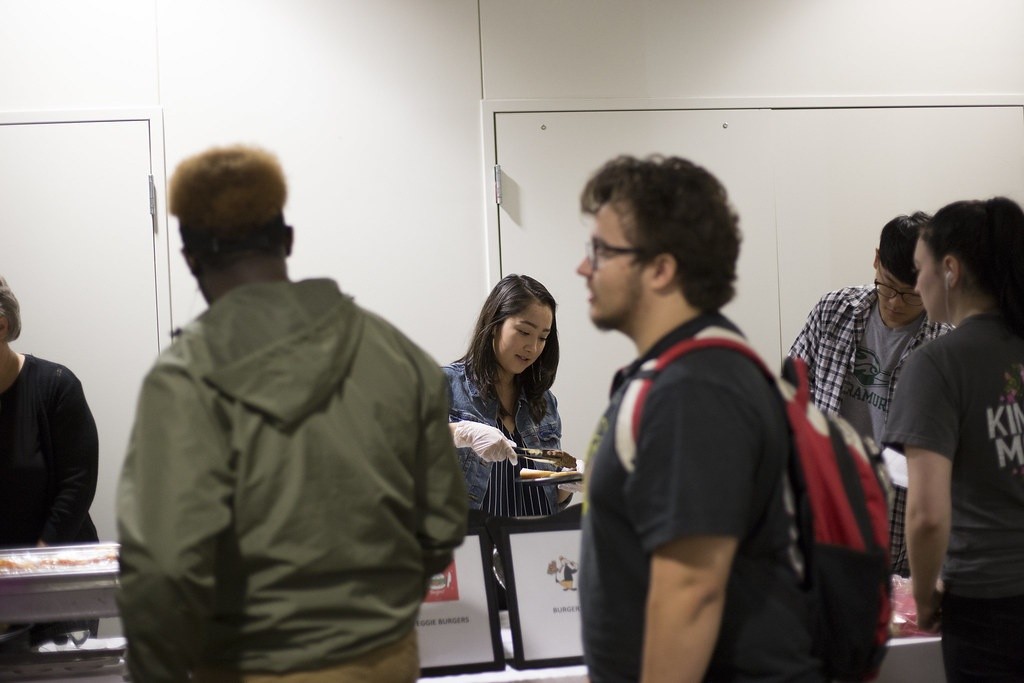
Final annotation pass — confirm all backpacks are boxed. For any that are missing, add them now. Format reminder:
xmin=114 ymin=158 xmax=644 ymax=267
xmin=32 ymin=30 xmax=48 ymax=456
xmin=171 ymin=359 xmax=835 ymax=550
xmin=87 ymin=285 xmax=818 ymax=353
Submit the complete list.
xmin=614 ymin=324 xmax=894 ymax=683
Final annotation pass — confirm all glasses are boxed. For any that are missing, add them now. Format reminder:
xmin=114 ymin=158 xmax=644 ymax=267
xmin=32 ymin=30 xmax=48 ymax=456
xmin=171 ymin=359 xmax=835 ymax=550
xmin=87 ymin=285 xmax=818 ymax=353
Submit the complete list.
xmin=874 ymin=256 xmax=924 ymax=307
xmin=588 ymin=235 xmax=659 ymax=270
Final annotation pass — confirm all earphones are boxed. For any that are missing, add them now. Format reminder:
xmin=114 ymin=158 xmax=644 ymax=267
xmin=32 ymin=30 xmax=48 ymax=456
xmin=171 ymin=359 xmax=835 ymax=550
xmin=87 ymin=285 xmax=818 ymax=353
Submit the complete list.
xmin=945 ymin=271 xmax=953 ymax=291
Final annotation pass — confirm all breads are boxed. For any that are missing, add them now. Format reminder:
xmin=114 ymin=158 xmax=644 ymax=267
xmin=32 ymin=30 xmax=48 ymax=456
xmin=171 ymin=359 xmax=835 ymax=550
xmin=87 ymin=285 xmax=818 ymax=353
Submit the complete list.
xmin=519 ymin=467 xmax=580 ymax=478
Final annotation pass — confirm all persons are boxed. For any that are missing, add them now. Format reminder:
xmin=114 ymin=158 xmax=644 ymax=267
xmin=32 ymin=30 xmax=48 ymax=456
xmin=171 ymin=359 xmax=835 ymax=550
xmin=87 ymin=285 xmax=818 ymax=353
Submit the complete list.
xmin=440 ymin=273 xmax=586 ymax=515
xmin=116 ymin=145 xmax=466 ymax=683
xmin=787 ymin=212 xmax=957 ymax=576
xmin=575 ymin=154 xmax=814 ymax=683
xmin=882 ymin=197 xmax=1024 ymax=682
xmin=0 ymin=279 xmax=99 ymax=645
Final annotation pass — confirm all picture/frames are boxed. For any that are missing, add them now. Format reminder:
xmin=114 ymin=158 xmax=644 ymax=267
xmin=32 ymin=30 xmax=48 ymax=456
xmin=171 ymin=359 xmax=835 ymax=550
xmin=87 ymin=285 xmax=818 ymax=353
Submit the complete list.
xmin=419 ymin=501 xmax=585 ymax=677
